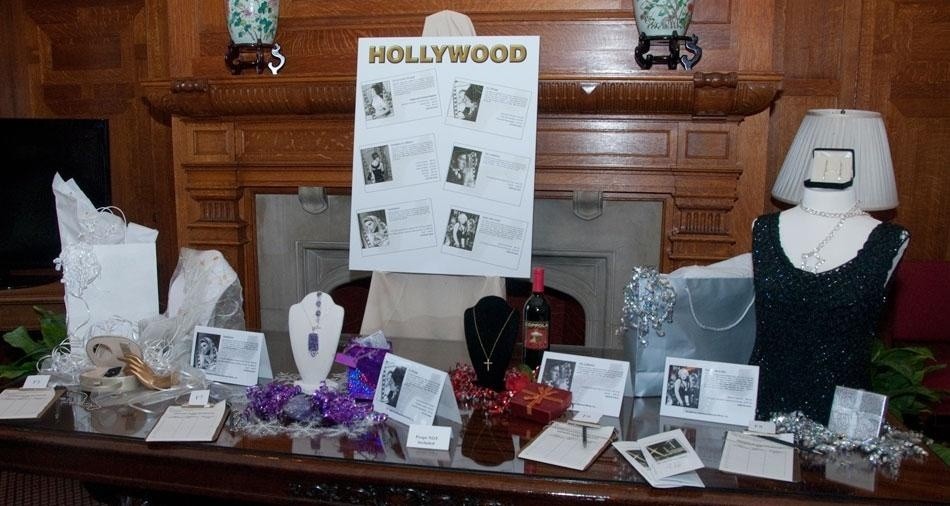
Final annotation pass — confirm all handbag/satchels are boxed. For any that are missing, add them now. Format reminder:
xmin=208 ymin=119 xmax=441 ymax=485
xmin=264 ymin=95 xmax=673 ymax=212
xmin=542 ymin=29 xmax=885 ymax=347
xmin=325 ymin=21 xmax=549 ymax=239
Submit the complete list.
xmin=62 ymin=207 xmax=159 ymax=354
xmin=622 ymin=275 xmax=759 ymax=398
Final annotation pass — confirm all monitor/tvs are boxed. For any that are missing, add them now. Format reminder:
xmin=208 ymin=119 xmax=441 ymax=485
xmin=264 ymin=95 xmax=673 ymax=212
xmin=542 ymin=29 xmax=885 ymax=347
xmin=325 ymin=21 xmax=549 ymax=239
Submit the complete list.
xmin=0 ymin=117 xmax=112 ymax=290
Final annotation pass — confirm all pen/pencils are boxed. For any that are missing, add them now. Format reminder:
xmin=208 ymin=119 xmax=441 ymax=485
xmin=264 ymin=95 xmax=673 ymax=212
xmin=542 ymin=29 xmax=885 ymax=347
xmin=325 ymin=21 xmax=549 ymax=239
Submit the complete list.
xmin=760 ymin=436 xmax=825 ymax=458
xmin=581 ymin=425 xmax=588 ymax=450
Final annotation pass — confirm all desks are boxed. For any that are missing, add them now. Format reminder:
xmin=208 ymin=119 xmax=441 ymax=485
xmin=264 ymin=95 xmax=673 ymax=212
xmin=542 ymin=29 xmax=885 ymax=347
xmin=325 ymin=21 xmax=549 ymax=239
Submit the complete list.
xmin=2 ymin=324 xmax=950 ymax=504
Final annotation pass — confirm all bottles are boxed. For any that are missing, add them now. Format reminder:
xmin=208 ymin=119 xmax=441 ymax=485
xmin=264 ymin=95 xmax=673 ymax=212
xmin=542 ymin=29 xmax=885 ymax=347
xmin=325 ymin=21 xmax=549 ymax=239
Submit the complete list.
xmin=521 ymin=266 xmax=551 ymax=371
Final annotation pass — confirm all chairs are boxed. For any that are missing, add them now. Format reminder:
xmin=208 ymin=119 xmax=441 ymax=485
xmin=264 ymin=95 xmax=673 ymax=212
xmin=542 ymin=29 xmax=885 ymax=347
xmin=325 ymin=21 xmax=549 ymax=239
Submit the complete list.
xmin=876 ymin=256 xmax=949 ymax=431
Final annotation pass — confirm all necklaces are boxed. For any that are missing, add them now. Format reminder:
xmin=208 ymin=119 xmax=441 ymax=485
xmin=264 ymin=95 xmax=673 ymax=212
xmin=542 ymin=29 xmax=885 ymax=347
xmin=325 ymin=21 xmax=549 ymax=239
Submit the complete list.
xmin=472 ymin=307 xmax=515 ymax=372
xmin=302 ymin=290 xmax=323 ymax=358
xmin=792 ymin=200 xmax=871 ymax=275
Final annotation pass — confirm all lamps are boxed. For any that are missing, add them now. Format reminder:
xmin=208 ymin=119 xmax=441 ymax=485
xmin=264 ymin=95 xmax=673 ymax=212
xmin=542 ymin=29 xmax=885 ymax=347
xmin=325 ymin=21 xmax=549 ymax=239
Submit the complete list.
xmin=769 ymin=105 xmax=903 ymax=216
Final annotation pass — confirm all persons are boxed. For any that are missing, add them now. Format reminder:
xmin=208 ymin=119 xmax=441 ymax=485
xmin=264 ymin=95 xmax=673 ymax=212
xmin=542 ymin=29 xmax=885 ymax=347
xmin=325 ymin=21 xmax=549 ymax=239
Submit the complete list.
xmin=116 ymin=351 xmax=180 ymax=391
xmin=198 ymin=338 xmax=217 ymax=374
xmin=447 ymin=213 xmax=476 ymax=249
xmin=447 ymin=151 xmax=467 ymax=184
xmin=385 ymin=367 xmax=406 ymax=407
xmin=541 ymin=360 xmax=571 ymax=391
xmin=459 ymin=84 xmax=482 ymax=121
xmin=749 ymin=149 xmax=912 ymax=426
xmin=367 ymin=85 xmax=391 ymax=119
xmin=368 ymin=152 xmax=386 ymax=183
xmin=667 ymin=368 xmax=701 ymax=410
xmin=363 ymin=215 xmax=386 ymax=247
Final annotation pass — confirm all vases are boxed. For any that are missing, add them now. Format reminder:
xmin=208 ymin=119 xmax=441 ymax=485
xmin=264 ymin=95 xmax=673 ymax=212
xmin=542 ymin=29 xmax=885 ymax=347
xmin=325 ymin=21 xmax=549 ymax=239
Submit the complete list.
xmin=631 ymin=0 xmax=695 ymax=37
xmin=225 ymin=0 xmax=279 ymax=48
